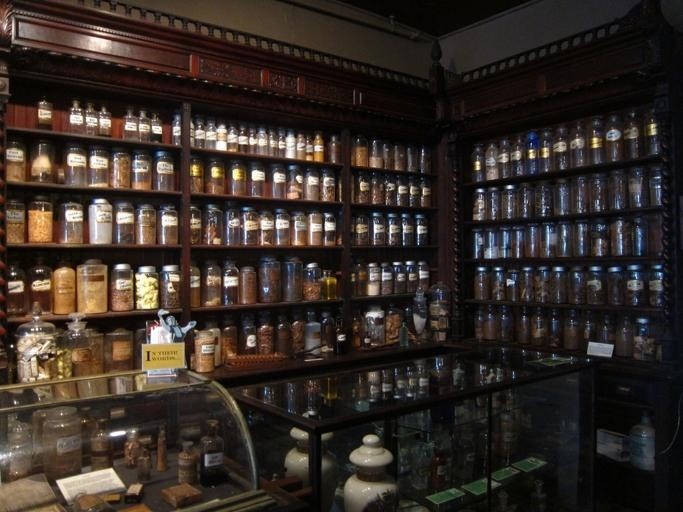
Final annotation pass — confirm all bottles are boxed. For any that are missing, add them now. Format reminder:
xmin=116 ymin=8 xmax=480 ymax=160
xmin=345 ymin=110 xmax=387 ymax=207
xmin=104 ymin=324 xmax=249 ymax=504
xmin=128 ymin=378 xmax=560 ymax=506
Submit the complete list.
xmin=150 ymin=112 xmax=165 ymax=143
xmin=189 ymin=114 xmax=341 ymax=166
xmin=67 ymin=100 xmax=84 ymax=133
xmin=188 ymin=153 xmax=340 ymax=205
xmin=353 ymin=168 xmax=432 ymax=210
xmin=349 ymin=297 xmax=433 ymax=354
xmin=5 ymin=421 xmax=33 ymax=481
xmin=136 ymin=435 xmax=157 ymax=485
xmin=138 ymin=110 xmax=150 ymax=140
xmin=79 ymin=406 xmax=95 ymax=459
xmin=124 ymin=427 xmax=140 ymax=468
xmin=83 ymin=102 xmax=99 ymax=135
xmin=97 ymin=106 xmax=113 ymax=136
xmin=352 ymin=257 xmax=431 ymax=297
xmin=472 ymin=304 xmax=671 ymax=365
xmin=5 ymin=252 xmax=183 ymax=314
xmin=177 ymin=442 xmax=197 ymax=484
xmin=472 ymin=163 xmax=663 ymax=222
xmin=35 ymin=96 xmax=53 ymax=129
xmin=172 ymin=111 xmax=181 ymax=145
xmin=120 ymin=107 xmax=138 ymax=140
xmin=193 ymin=306 xmax=348 ymax=375
xmin=352 ymin=132 xmax=433 ymax=175
xmin=391 ymin=428 xmax=524 ymax=498
xmin=469 ymin=104 xmax=662 ymax=184
xmin=0 ymin=137 xmax=178 ymax=193
xmin=197 ymin=420 xmax=222 ymax=486
xmin=189 ymin=254 xmax=340 ymax=309
xmin=89 ymin=417 xmax=113 ymax=471
xmin=190 ymin=198 xmax=338 ymax=249
xmin=155 ymin=425 xmax=170 ymax=473
xmin=468 ymin=263 xmax=666 ymax=309
xmin=3 ymin=187 xmax=179 ymax=249
xmin=472 ymin=215 xmax=662 ymax=261
xmin=255 ymin=351 xmax=521 ymax=426
xmin=349 ymin=208 xmax=430 ymax=247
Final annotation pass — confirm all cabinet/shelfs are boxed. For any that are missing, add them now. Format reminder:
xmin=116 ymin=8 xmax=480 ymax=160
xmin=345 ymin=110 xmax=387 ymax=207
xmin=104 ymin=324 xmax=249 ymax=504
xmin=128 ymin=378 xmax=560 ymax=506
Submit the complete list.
xmin=0 ymin=44 xmax=189 ymax=386
xmin=452 ymin=64 xmax=682 ymax=477
xmin=1 ymin=371 xmax=280 ymax=512
xmin=225 ymin=345 xmax=608 ymax=512
xmin=346 ymin=101 xmax=453 ymax=364
xmin=185 ymin=71 xmax=348 ymax=379
